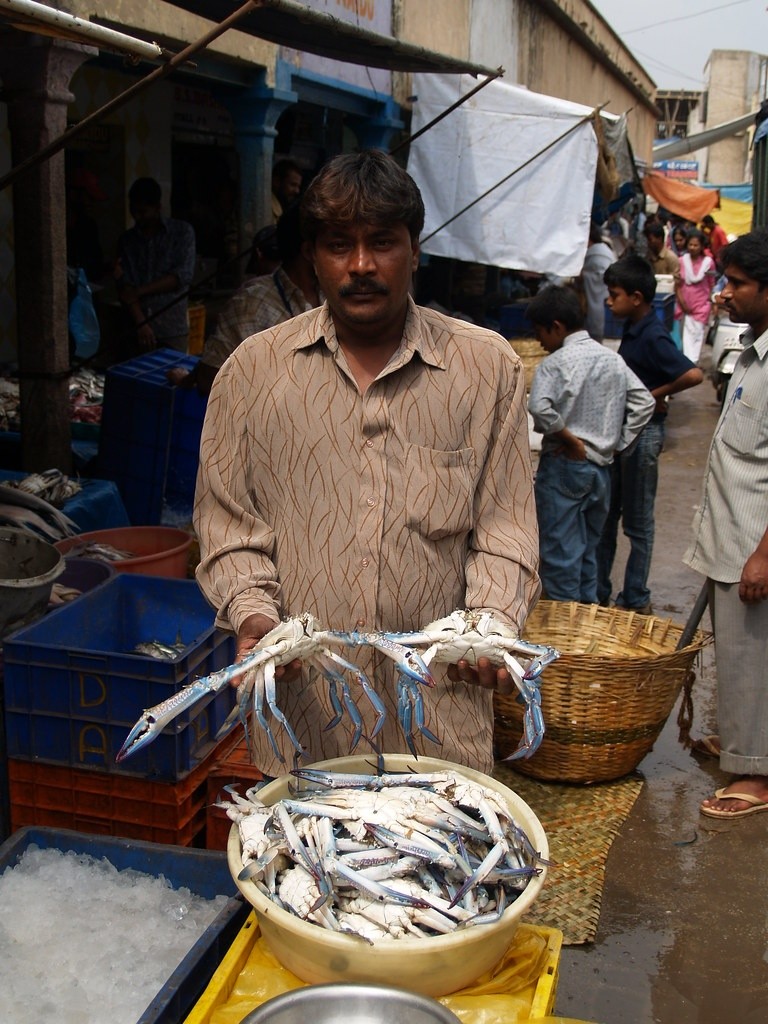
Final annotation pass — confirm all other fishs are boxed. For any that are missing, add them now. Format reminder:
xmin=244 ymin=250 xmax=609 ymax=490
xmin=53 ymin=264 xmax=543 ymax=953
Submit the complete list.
xmin=0 ymin=485 xmax=82 ymax=540
xmin=0 ymin=504 xmax=64 ymax=541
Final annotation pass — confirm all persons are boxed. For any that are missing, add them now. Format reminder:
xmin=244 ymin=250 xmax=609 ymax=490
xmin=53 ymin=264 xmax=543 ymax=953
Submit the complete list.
xmin=115 ymin=177 xmax=196 ymax=353
xmin=192 ymin=152 xmax=542 ymax=782
xmin=271 ymin=159 xmax=303 ymax=224
xmin=252 ymin=224 xmax=285 ymax=274
xmin=583 ymin=222 xmax=617 ymax=344
xmin=596 ymin=255 xmax=705 ymax=615
xmin=680 ymin=225 xmax=768 ymax=820
xmin=701 ymin=215 xmax=728 ymax=261
xmin=642 ymin=225 xmax=680 ymax=282
xmin=603 ymin=203 xmax=690 ymax=256
xmin=522 ymin=284 xmax=656 ymax=604
xmin=194 ymin=196 xmax=327 ymax=397
xmin=676 ymin=231 xmax=718 ymax=364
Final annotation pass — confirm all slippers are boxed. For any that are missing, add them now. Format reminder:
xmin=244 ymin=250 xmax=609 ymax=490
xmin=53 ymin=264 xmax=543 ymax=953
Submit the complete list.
xmin=699 ymin=780 xmax=768 ymax=819
xmin=691 ymin=736 xmax=721 ymax=756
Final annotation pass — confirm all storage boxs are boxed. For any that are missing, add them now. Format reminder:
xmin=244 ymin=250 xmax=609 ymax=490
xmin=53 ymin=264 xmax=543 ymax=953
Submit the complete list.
xmin=182 ymin=910 xmax=567 ymax=1024
xmin=100 ymin=346 xmax=210 ymax=531
xmin=500 ymin=302 xmax=533 ymax=330
xmin=197 ymin=714 xmax=262 ymax=851
xmin=0 ymin=825 xmax=245 ymax=1024
xmin=6 ymin=764 xmax=208 ymax=862
xmin=0 ymin=571 xmax=236 ymax=780
xmin=601 ymin=289 xmax=676 ymax=340
xmin=186 ymin=300 xmax=207 ymax=355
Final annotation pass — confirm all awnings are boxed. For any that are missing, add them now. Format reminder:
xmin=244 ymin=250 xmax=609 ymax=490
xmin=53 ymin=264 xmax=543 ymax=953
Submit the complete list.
xmin=0 ymin=0 xmax=506 ymax=208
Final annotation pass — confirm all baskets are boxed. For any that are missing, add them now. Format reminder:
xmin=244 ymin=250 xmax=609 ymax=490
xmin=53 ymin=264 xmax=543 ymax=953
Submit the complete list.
xmin=493 ymin=602 xmax=710 ymax=783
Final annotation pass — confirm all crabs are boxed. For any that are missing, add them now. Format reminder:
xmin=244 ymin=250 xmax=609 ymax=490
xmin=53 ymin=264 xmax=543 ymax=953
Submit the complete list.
xmin=374 ymin=609 xmax=562 ymax=763
xmin=115 ymin=613 xmax=438 ymax=764
xmin=210 ymin=733 xmax=560 ymax=946
xmin=0 ymin=468 xmax=83 ymax=502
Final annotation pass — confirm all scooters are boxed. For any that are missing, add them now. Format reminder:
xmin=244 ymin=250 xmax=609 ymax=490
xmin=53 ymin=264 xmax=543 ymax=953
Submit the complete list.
xmin=708 ymin=299 xmax=749 ymax=411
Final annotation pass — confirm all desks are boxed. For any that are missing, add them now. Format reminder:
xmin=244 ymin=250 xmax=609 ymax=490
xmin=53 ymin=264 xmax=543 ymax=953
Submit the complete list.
xmin=0 ymin=470 xmax=130 ymax=540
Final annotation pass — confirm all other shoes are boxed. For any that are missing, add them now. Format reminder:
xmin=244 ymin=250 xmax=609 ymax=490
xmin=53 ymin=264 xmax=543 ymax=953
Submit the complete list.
xmin=612 ymin=603 xmax=652 ymax=615
